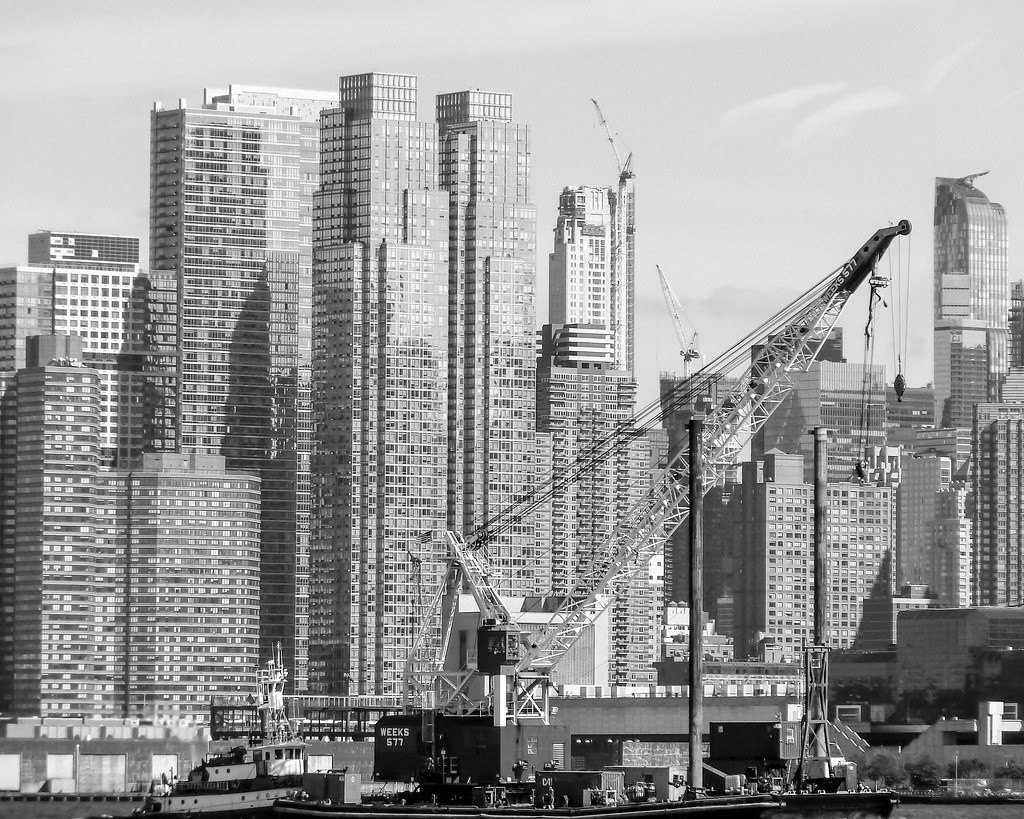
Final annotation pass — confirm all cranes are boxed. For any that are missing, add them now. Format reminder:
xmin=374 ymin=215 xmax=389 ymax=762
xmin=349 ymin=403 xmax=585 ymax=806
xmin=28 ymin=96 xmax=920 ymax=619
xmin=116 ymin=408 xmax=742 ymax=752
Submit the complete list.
xmin=375 ymin=217 xmax=914 ymax=798
xmin=655 ymin=264 xmax=701 ymax=379
xmin=589 ymin=98 xmax=636 ymax=179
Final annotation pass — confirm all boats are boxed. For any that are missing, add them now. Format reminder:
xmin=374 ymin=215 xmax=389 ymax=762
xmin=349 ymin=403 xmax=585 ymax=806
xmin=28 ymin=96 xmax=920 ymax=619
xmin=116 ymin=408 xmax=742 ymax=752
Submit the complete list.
xmin=132 ymin=639 xmax=311 ymax=815
